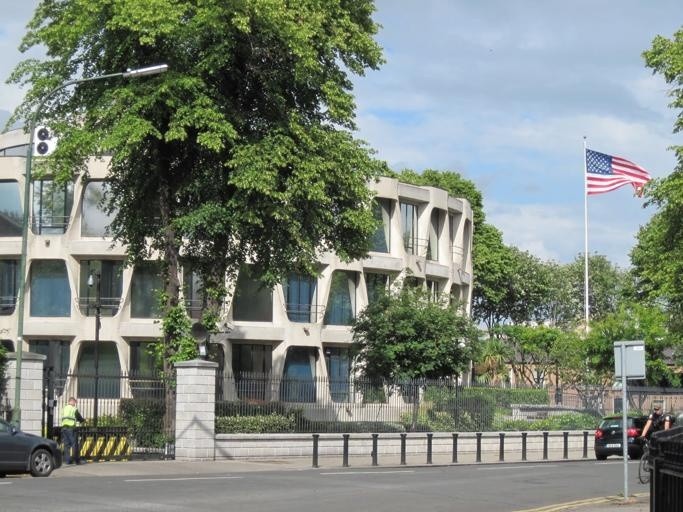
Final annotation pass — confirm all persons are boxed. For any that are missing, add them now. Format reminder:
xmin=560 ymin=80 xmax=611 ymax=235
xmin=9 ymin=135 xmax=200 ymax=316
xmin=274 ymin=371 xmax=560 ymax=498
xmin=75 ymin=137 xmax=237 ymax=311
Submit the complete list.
xmin=640 ymin=402 xmax=671 ymax=437
xmin=62 ymin=397 xmax=85 ymax=465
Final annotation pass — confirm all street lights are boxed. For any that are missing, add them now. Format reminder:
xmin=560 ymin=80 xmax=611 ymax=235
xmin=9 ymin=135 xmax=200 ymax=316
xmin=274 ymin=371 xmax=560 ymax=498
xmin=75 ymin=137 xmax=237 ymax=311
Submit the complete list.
xmin=12 ymin=64 xmax=168 ymax=424
xmin=88 ymin=271 xmax=99 ymax=426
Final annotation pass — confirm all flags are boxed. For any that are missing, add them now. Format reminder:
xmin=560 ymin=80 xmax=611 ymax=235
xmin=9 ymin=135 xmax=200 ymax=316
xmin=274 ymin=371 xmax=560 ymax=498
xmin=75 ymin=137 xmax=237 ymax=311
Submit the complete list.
xmin=586 ymin=148 xmax=652 ymax=197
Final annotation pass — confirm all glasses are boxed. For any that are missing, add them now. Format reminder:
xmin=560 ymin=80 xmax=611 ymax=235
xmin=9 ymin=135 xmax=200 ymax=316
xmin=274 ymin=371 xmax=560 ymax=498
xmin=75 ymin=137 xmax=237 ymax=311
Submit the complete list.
xmin=654 ymin=408 xmax=659 ymax=411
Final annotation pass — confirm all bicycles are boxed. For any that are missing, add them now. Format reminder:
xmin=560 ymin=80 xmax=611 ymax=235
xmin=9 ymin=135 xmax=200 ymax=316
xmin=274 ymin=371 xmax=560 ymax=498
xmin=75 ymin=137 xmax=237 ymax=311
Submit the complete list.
xmin=639 ymin=434 xmax=653 ymax=484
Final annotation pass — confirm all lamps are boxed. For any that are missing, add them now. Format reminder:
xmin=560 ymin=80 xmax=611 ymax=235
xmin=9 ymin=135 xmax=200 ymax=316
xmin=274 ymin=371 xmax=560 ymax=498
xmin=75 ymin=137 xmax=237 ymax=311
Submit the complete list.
xmin=325 ymin=348 xmax=331 ymax=354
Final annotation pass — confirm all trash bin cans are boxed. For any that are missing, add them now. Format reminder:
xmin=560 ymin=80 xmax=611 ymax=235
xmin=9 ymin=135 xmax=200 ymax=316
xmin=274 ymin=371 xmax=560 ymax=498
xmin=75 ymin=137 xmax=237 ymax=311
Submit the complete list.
xmin=646 ymin=426 xmax=683 ymax=512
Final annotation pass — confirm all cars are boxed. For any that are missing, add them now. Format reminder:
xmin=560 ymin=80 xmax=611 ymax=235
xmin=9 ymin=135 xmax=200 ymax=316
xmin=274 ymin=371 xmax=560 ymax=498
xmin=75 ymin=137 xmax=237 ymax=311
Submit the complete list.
xmin=0 ymin=419 xmax=63 ymax=477
xmin=594 ymin=415 xmax=650 ymax=460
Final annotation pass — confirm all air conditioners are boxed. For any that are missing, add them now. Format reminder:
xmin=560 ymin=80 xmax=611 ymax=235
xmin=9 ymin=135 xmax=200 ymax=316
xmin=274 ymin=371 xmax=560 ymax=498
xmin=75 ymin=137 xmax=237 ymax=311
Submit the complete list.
xmin=33 ymin=125 xmax=57 ymax=156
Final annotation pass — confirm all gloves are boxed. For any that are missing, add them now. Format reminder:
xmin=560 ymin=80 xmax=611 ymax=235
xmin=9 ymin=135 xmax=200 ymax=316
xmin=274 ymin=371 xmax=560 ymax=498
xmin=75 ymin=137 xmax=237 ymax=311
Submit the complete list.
xmin=636 ymin=436 xmax=645 ymax=443
